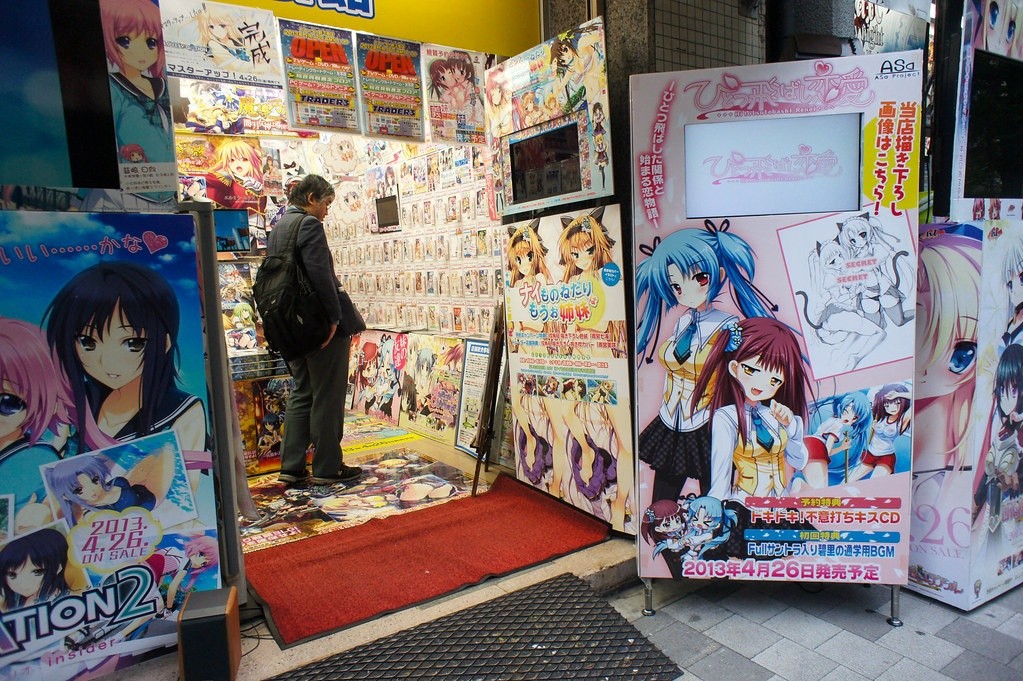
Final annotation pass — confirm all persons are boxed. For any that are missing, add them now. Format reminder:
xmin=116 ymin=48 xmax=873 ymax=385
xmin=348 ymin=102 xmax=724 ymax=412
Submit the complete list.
xmin=266 ymin=174 xmax=363 ymax=485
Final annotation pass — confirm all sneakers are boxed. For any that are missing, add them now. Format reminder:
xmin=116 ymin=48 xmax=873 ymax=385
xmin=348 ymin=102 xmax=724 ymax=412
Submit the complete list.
xmin=311 ymin=461 xmax=362 ymax=484
xmin=277 ymin=468 xmax=310 ymax=482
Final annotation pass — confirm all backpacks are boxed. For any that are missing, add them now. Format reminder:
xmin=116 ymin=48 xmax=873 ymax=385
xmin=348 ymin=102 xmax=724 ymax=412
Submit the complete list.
xmin=251 ymin=212 xmax=323 ymax=362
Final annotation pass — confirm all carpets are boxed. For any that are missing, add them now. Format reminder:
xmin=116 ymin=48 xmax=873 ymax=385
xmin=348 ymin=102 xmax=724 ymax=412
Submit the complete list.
xmin=243 ymin=470 xmax=613 ymax=651
